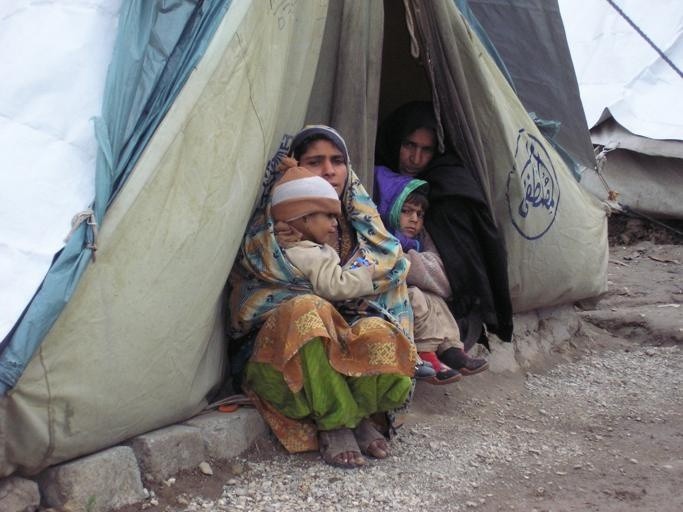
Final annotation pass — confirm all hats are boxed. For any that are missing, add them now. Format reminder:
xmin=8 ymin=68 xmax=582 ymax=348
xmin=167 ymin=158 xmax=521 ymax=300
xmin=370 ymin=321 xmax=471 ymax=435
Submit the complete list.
xmin=271 ymin=158 xmax=342 ymax=221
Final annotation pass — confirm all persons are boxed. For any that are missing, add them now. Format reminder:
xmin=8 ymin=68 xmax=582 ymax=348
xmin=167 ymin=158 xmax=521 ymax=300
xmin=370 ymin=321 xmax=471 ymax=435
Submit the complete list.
xmin=374 ymin=101 xmax=513 ymax=386
xmin=228 ymin=123 xmax=420 ymax=470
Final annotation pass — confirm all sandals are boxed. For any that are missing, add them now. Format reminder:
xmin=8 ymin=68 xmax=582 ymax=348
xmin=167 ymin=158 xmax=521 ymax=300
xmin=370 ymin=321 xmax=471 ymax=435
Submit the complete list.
xmin=355 ymin=419 xmax=392 ymax=457
xmin=319 ymin=428 xmax=364 ymax=468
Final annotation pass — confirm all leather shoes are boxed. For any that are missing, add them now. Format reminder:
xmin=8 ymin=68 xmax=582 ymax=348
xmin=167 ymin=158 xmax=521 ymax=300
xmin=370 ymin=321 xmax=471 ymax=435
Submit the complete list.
xmin=416 ymin=361 xmax=436 ymax=379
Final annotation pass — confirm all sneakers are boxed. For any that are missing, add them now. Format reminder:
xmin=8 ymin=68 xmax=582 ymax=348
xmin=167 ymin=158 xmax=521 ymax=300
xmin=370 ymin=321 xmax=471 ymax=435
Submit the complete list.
xmin=439 ymin=347 xmax=488 ymax=375
xmin=419 ymin=352 xmax=462 ymax=385
